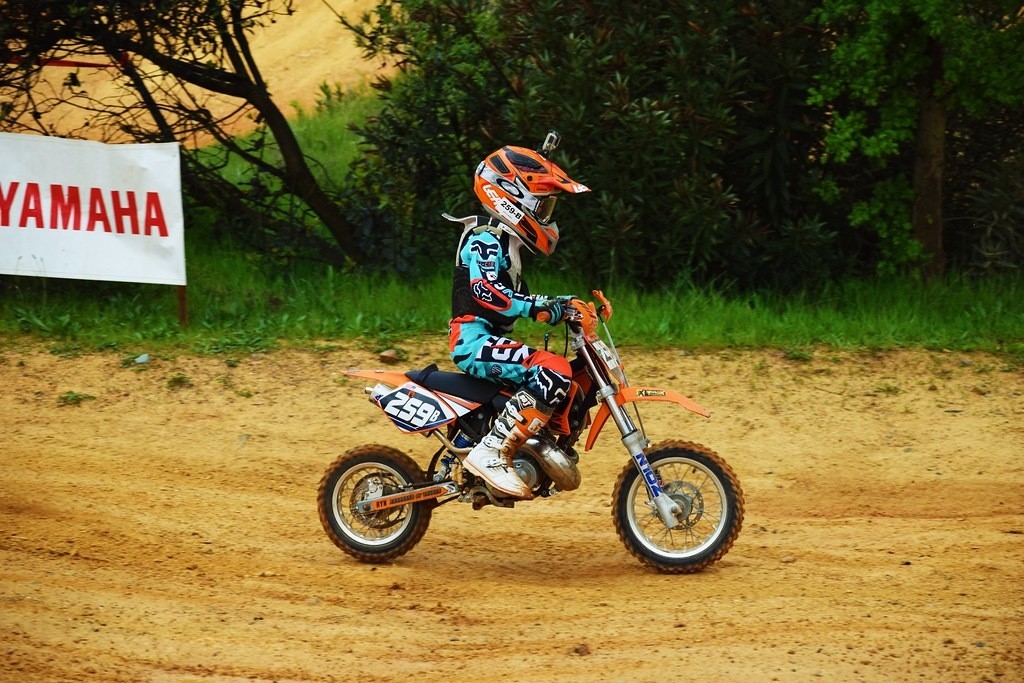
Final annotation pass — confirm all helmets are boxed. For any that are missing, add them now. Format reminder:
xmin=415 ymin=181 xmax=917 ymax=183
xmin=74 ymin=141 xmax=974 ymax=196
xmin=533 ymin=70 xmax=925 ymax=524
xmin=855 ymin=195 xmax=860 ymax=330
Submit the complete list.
xmin=473 ymin=145 xmax=593 ymax=257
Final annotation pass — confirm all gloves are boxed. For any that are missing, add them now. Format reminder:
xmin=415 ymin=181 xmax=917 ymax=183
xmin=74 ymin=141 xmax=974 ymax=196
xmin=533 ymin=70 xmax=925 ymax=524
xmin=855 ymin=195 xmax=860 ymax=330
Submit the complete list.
xmin=529 ymin=300 xmax=567 ymax=326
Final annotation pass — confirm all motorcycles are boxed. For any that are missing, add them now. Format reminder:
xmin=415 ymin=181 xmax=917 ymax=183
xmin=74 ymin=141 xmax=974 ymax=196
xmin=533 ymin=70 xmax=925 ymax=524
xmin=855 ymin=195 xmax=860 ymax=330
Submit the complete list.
xmin=317 ymin=288 xmax=746 ymax=572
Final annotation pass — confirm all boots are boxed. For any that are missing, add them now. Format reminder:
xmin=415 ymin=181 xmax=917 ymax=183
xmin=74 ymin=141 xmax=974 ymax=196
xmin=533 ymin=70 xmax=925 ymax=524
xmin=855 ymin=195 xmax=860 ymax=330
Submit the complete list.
xmin=461 ymin=385 xmax=556 ymax=500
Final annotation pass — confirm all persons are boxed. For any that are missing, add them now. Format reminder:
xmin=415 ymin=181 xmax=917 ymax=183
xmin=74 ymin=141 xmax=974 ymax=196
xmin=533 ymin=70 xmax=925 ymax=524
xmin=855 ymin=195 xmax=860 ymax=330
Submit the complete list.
xmin=447 ymin=145 xmax=597 ymax=497
